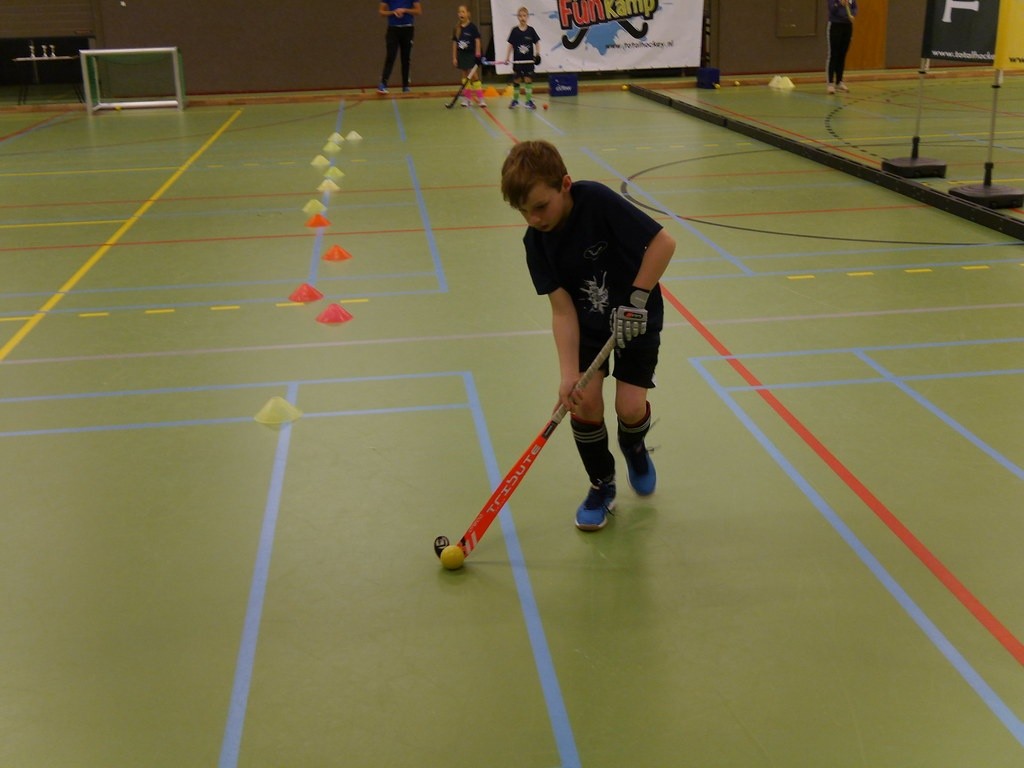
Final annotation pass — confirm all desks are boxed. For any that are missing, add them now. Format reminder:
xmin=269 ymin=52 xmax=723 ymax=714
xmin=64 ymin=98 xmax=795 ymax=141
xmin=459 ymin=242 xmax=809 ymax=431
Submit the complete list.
xmin=12 ymin=57 xmax=89 ymax=106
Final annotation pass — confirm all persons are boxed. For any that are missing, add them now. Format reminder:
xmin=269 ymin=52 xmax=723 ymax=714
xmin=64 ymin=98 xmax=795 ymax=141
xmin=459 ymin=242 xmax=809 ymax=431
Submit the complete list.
xmin=826 ymin=0 xmax=857 ymax=93
xmin=451 ymin=5 xmax=486 ymax=107
xmin=499 ymin=139 xmax=675 ymax=530
xmin=376 ymin=0 xmax=422 ymax=94
xmin=506 ymin=7 xmax=541 ymax=109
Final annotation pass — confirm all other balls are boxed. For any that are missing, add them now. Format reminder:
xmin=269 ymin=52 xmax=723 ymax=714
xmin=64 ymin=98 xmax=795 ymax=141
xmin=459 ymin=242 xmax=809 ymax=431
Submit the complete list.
xmin=734 ymin=81 xmax=741 ymax=86
xmin=543 ymin=103 xmax=549 ymax=109
xmin=715 ymin=84 xmax=721 ymax=89
xmin=440 ymin=545 xmax=465 ymax=570
xmin=622 ymin=85 xmax=628 ymax=90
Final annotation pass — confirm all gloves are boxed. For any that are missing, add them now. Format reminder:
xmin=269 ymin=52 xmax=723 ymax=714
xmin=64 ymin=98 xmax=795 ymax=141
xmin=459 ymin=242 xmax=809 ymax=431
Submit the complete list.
xmin=475 ymin=57 xmax=481 ymax=66
xmin=534 ymin=55 xmax=541 ymax=65
xmin=609 ymin=287 xmax=650 ymax=348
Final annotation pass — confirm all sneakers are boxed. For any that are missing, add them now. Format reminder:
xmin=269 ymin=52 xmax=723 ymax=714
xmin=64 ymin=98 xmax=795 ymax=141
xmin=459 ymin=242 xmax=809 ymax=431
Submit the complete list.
xmin=834 ymin=82 xmax=850 ymax=93
xmin=524 ymin=101 xmax=536 ymax=109
xmin=828 ymin=83 xmax=836 ymax=93
xmin=625 ymin=446 xmax=657 ymax=496
xmin=508 ymin=100 xmax=519 ymax=108
xmin=576 ymin=481 xmax=618 ymax=530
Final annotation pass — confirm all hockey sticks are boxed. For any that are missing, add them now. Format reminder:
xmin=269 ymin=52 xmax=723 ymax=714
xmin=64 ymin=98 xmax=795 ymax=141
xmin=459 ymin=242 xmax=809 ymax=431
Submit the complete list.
xmin=481 ymin=56 xmax=541 ymax=66
xmin=443 ymin=59 xmax=481 ymax=109
xmin=433 ymin=333 xmax=618 ymax=562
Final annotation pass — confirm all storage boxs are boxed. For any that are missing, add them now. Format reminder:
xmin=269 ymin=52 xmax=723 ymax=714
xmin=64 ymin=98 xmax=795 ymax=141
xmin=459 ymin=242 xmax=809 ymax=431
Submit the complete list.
xmin=549 ymin=73 xmax=579 ymax=97
xmin=695 ymin=67 xmax=720 ymax=90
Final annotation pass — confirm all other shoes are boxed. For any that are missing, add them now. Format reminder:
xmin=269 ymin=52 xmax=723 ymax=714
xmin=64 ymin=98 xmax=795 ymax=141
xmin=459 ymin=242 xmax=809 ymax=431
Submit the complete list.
xmin=403 ymin=87 xmax=409 ymax=92
xmin=377 ymin=87 xmax=389 ymax=94
xmin=461 ymin=99 xmax=473 ymax=106
xmin=479 ymin=102 xmax=487 ymax=107
